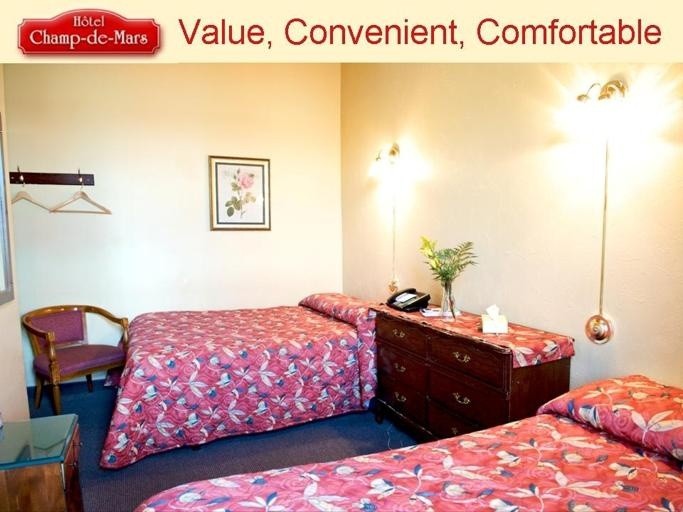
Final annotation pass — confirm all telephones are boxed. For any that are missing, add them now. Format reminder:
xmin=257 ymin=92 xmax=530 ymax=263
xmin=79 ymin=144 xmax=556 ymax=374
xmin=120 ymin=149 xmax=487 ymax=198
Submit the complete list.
xmin=386 ymin=288 xmax=430 ymax=312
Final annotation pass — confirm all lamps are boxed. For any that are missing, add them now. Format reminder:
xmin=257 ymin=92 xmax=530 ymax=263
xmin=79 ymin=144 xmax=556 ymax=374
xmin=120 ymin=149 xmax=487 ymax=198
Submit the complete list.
xmin=374 ymin=141 xmax=410 ymax=300
xmin=575 ymin=76 xmax=630 ymax=346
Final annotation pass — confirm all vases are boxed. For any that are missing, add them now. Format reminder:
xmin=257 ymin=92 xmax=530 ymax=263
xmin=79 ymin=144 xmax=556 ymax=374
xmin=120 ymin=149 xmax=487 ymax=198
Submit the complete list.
xmin=441 ymin=282 xmax=461 ymax=316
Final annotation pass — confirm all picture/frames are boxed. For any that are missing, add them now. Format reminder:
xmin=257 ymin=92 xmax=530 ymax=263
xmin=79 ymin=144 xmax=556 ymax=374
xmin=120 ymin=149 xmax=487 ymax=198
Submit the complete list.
xmin=208 ymin=155 xmax=272 ymax=229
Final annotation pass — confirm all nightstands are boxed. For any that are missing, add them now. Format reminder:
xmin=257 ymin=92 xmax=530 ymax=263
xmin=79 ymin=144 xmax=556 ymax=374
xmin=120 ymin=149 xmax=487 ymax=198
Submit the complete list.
xmin=369 ymin=304 xmax=576 ymax=444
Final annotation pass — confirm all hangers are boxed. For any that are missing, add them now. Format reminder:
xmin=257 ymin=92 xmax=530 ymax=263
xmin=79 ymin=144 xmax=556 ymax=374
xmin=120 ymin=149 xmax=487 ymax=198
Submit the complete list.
xmin=10 ymin=166 xmax=50 ymax=212
xmin=48 ymin=167 xmax=115 ymax=215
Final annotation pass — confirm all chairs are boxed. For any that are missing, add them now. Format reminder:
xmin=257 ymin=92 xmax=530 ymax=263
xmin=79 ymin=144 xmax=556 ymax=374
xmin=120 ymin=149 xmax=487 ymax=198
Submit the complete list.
xmin=22 ymin=305 xmax=131 ymax=415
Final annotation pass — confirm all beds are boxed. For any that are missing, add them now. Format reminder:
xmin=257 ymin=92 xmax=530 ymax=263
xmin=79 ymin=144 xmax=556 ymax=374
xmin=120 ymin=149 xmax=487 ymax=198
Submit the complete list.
xmin=98 ymin=292 xmax=379 ymax=470
xmin=132 ymin=375 xmax=683 ymax=512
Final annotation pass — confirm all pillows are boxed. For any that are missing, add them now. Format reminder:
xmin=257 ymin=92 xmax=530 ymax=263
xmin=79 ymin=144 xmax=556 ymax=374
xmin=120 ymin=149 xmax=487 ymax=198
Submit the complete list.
xmin=298 ymin=292 xmax=379 ymax=332
xmin=551 ymin=375 xmax=683 ymax=460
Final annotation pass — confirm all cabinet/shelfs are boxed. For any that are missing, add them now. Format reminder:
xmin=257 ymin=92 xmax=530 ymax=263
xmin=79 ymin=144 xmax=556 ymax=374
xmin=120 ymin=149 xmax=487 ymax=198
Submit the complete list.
xmin=0 ymin=414 xmax=83 ymax=512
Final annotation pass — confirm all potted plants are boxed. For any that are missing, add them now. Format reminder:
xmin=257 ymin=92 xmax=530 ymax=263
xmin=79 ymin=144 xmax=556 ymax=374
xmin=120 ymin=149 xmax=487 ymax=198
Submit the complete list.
xmin=420 ymin=236 xmax=479 ymax=318
